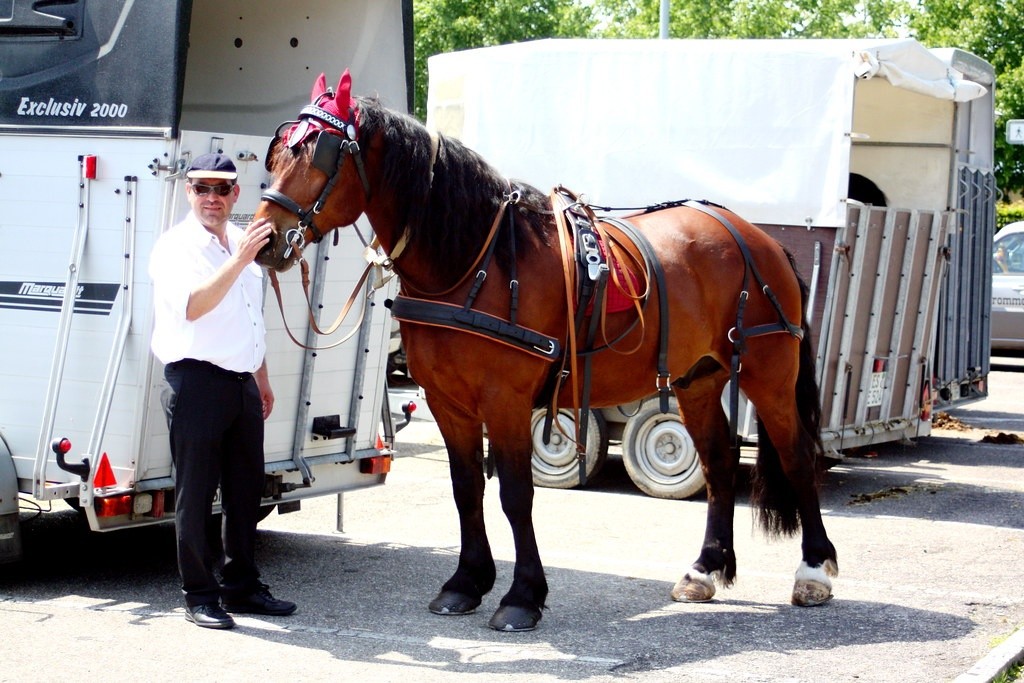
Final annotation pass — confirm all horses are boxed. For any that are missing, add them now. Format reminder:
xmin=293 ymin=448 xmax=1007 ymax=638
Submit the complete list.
xmin=248 ymin=66 xmax=839 ymax=632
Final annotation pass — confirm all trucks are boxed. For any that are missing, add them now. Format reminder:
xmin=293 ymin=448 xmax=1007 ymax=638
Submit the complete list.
xmin=0 ymin=0 xmax=414 ymax=580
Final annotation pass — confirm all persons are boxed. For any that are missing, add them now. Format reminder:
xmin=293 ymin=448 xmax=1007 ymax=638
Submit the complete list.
xmin=151 ymin=154 xmax=298 ymax=628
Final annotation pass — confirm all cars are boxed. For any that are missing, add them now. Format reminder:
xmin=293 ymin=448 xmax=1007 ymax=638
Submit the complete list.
xmin=988 ymin=222 xmax=1024 ymax=359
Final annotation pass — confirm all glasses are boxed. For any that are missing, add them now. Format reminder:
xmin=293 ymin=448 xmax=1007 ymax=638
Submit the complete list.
xmin=188 ymin=183 xmax=236 ymax=196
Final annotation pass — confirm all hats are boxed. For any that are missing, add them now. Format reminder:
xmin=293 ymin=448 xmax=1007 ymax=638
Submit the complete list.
xmin=186 ymin=153 xmax=238 ymax=180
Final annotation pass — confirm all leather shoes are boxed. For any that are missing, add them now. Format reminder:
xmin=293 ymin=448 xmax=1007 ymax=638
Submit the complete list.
xmin=184 ymin=601 xmax=235 ymax=628
xmin=218 ymin=579 xmax=297 ymax=615
